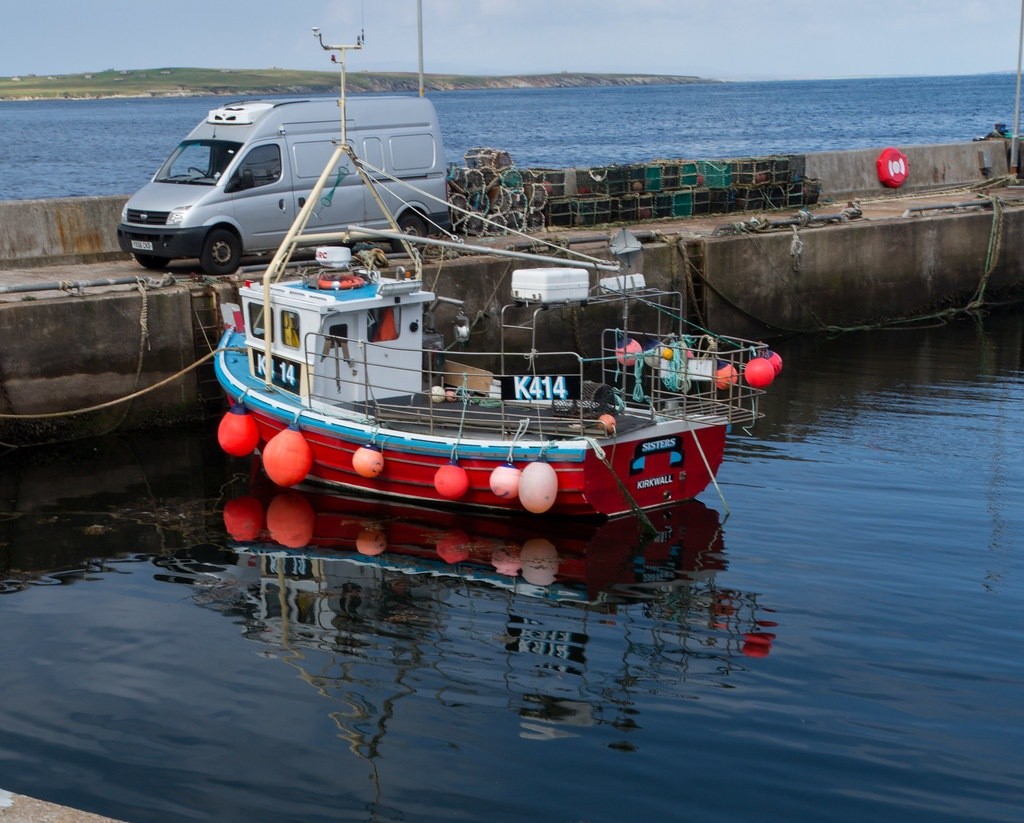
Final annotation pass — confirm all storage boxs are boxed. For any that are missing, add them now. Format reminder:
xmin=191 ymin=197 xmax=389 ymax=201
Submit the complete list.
xmin=512 ymin=267 xmax=589 ymax=300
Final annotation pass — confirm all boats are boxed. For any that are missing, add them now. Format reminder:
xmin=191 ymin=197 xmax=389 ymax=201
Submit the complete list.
xmin=214 ymin=27 xmax=783 ymax=518
xmin=219 ymin=473 xmax=777 ymax=802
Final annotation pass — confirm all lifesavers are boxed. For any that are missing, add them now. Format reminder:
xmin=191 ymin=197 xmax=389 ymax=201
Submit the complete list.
xmin=308 ymin=273 xmax=365 ymax=290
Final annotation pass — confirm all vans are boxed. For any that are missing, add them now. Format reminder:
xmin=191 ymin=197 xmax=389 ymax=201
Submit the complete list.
xmin=118 ymin=95 xmax=452 ymax=276
